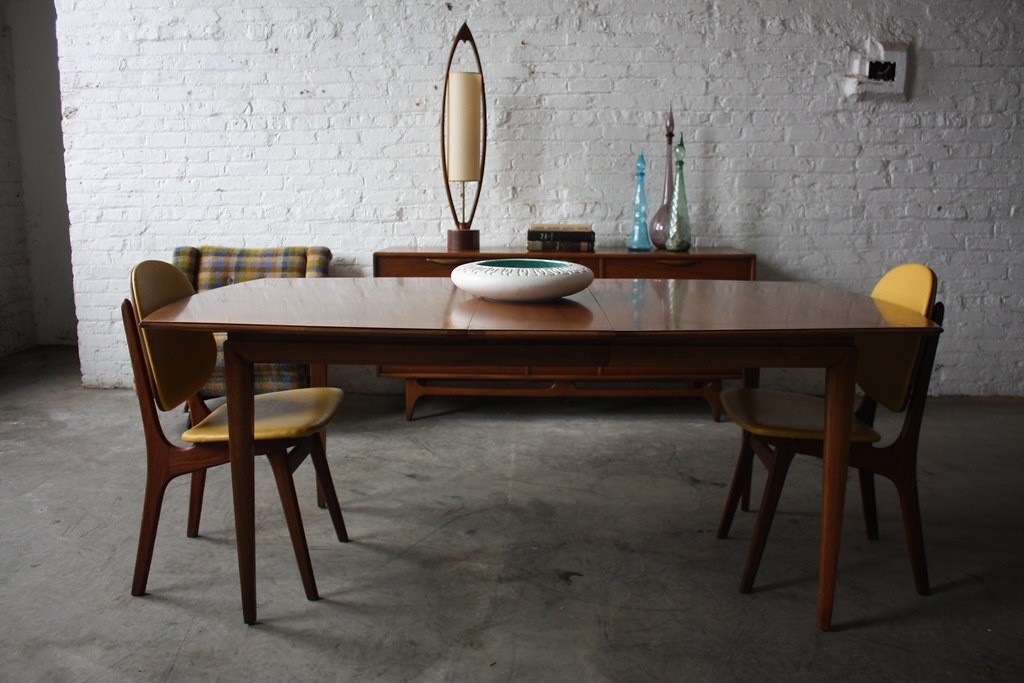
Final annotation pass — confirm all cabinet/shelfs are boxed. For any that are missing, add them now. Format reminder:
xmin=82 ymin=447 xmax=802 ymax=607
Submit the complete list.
xmin=373 ymin=248 xmax=757 ymax=423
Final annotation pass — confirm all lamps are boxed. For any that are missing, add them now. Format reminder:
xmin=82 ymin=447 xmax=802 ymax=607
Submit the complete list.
xmin=440 ymin=22 xmax=487 ymax=252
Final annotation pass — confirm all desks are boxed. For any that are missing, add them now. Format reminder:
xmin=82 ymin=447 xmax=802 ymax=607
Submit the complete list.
xmin=138 ymin=275 xmax=945 ymax=631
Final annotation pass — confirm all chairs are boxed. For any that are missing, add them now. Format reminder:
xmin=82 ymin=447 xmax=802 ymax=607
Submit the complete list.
xmin=172 ymin=244 xmax=333 ymax=431
xmin=715 ymin=262 xmax=946 ymax=597
xmin=121 ymin=260 xmax=352 ymax=600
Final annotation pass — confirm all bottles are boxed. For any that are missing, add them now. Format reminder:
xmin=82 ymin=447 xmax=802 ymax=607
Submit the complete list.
xmin=629 ymin=107 xmax=691 ymax=252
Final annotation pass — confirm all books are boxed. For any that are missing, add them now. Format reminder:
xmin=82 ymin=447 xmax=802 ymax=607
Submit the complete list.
xmin=527 ymin=223 xmax=596 ymax=253
